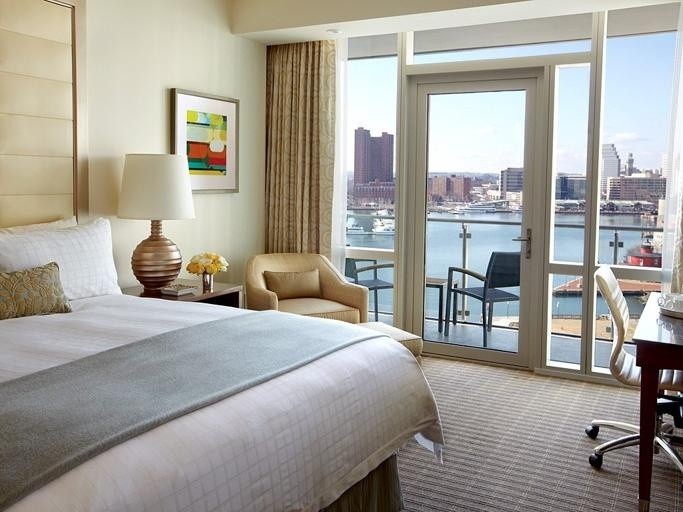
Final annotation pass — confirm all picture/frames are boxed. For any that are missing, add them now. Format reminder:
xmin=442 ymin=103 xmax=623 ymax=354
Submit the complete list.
xmin=170 ymin=88 xmax=239 ymax=194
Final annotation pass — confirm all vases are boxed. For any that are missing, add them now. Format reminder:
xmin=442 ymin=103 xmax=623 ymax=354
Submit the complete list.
xmin=202 ymin=273 xmax=214 ymax=290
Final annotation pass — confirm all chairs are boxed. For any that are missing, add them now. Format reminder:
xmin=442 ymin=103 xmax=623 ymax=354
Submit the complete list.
xmin=344 ymin=244 xmax=394 ymax=322
xmin=444 ymin=252 xmax=519 ymax=347
xmin=584 ymin=266 xmax=683 ymax=473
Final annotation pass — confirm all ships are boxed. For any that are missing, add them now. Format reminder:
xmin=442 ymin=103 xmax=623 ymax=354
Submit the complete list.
xmin=345 ymin=215 xmax=394 ymax=235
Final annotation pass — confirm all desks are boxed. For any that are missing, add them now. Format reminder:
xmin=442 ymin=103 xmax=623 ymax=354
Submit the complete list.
xmin=632 ymin=291 xmax=683 ymax=512
xmin=425 ymin=278 xmax=458 ymax=332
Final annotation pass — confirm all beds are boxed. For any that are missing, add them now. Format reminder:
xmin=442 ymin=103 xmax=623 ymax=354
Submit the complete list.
xmin=0 ymin=214 xmax=444 ymax=512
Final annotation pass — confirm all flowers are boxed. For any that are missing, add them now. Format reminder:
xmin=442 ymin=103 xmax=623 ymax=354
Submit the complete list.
xmin=186 ymin=252 xmax=229 ymax=276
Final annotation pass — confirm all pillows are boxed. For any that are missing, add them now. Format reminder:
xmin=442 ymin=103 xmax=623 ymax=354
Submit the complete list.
xmin=0 ymin=215 xmax=78 ymax=235
xmin=0 ymin=262 xmax=73 ymax=321
xmin=264 ymin=269 xmax=322 ymax=300
xmin=1 ymin=217 xmax=122 ymax=301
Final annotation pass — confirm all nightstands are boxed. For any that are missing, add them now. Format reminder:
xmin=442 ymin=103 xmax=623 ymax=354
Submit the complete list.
xmin=122 ymin=278 xmax=244 ymax=309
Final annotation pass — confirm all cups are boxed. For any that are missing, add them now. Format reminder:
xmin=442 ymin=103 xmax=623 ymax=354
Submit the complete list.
xmin=664 ymin=297 xmax=683 ymax=312
xmin=656 ymin=292 xmax=679 ymax=308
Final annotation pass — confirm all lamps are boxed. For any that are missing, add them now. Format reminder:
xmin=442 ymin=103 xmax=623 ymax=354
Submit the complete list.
xmin=117 ymin=154 xmax=196 ymax=291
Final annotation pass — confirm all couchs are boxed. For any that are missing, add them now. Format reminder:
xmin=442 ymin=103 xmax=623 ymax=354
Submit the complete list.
xmin=246 ymin=253 xmax=369 ymax=323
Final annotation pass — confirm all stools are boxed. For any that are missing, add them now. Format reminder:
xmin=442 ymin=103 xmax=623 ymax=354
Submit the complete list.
xmin=358 ymin=322 xmax=423 ymax=370
